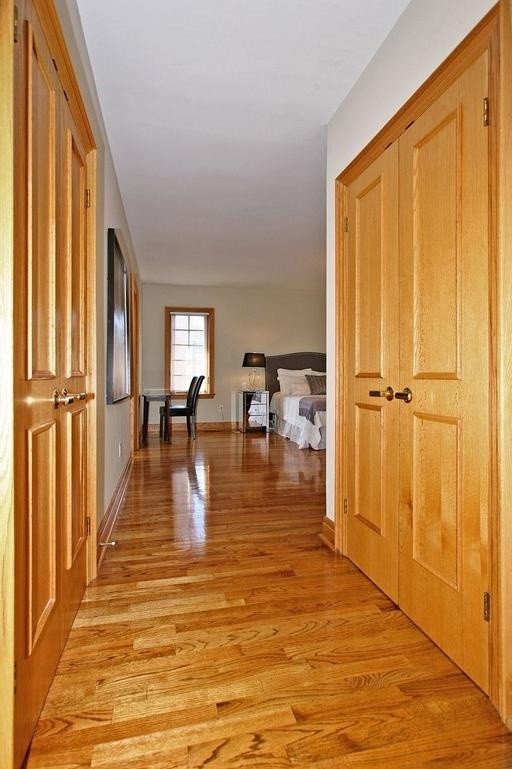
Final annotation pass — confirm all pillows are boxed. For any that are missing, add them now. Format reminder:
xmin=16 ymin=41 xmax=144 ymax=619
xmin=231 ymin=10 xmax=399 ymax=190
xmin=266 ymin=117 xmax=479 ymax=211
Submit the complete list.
xmin=275 ymin=367 xmax=327 ymax=397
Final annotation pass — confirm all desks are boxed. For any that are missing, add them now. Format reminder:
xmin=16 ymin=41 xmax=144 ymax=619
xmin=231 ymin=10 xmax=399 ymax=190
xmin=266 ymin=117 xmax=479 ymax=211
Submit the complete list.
xmin=140 ymin=389 xmax=175 ymax=446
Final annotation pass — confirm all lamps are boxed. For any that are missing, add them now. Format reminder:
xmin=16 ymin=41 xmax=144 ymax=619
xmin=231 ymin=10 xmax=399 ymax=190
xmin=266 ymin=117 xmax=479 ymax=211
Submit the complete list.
xmin=240 ymin=352 xmax=267 ymax=392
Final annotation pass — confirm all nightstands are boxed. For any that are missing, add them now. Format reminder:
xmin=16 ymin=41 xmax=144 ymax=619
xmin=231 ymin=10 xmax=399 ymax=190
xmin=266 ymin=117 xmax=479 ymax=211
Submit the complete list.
xmin=237 ymin=392 xmax=270 ymax=432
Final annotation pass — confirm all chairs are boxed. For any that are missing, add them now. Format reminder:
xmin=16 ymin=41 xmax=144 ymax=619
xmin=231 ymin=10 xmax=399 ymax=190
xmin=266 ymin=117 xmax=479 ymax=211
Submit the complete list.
xmin=158 ymin=375 xmax=205 ymax=443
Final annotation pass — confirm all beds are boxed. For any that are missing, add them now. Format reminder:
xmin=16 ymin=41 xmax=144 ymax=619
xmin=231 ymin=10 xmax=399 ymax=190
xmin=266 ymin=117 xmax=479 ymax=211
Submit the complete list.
xmin=265 ymin=353 xmax=326 ymax=451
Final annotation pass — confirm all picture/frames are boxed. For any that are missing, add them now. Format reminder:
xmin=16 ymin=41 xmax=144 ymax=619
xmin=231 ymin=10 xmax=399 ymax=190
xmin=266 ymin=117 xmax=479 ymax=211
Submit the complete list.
xmin=106 ymin=227 xmax=132 ymax=404
